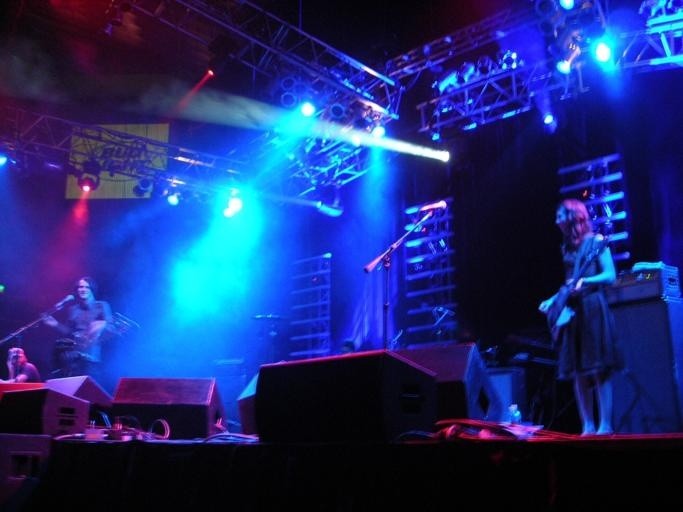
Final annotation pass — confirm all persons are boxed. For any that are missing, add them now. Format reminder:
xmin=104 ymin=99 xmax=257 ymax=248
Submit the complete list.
xmin=538 ymin=199 xmax=616 ymax=436
xmin=0 ymin=347 xmax=42 ymax=384
xmin=45 ymin=276 xmax=113 ymax=380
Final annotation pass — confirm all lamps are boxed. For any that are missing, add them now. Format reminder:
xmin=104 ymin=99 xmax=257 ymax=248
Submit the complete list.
xmin=555 ymin=42 xmax=581 ymax=75
xmin=76 ymin=158 xmax=101 ymax=192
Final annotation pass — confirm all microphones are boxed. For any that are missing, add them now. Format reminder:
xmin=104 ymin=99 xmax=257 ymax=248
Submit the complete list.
xmin=417 ymin=199 xmax=448 ymax=211
xmin=54 ymin=293 xmax=73 ymax=310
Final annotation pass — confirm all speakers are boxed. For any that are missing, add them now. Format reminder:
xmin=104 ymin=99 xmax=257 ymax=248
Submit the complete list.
xmin=0 ymin=388 xmax=92 ymax=435
xmin=44 ymin=373 xmax=112 ymax=411
xmin=112 ymin=377 xmax=228 ymax=435
xmin=254 ymin=338 xmax=505 ymax=433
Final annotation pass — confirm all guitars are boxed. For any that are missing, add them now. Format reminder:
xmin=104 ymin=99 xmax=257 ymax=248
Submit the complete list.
xmin=547 ymin=217 xmax=613 ymax=346
